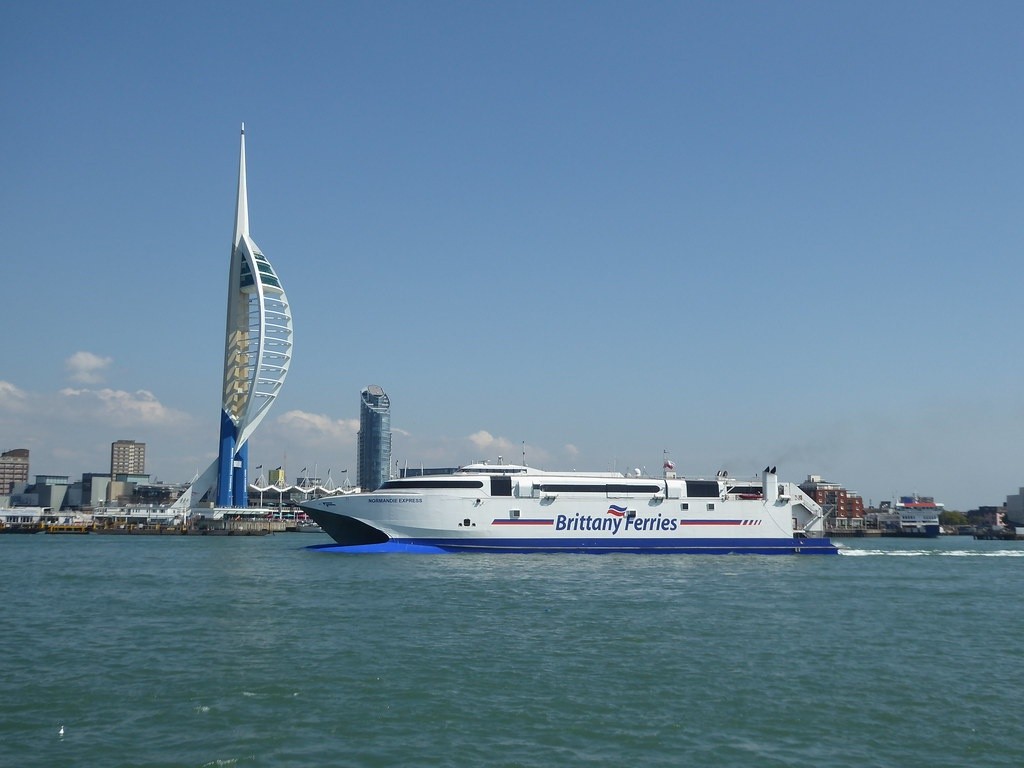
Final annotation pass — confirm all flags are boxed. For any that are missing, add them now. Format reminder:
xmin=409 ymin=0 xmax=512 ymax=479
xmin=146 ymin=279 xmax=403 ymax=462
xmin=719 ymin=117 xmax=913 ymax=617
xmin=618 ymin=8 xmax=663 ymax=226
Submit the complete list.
xmin=341 ymin=470 xmax=347 ymax=472
xmin=301 ymin=468 xmax=306 ymax=472
xmin=256 ymin=465 xmax=262 ymax=469
xmin=276 ymin=466 xmax=281 ymax=470
xmin=664 ymin=460 xmax=675 ymax=471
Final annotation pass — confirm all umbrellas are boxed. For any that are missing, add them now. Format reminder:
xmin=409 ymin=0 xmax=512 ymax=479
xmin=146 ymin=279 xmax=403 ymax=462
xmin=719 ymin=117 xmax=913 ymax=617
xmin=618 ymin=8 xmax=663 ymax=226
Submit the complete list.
xmin=283 ymin=514 xmax=294 ymax=519
xmin=299 ymin=514 xmax=309 ymax=520
xmin=267 ymin=514 xmax=280 ymax=518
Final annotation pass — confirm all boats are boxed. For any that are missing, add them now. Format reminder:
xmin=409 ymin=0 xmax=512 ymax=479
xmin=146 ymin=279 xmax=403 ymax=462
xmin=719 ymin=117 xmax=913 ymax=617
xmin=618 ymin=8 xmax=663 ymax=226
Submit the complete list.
xmin=0 ymin=521 xmax=269 ymax=536
xmin=292 ymin=441 xmax=841 ymax=555
xmin=739 ymin=491 xmax=763 ymax=499
